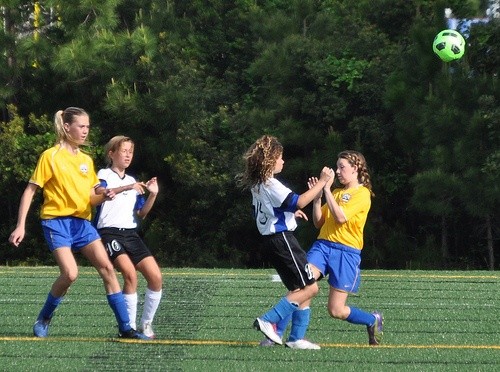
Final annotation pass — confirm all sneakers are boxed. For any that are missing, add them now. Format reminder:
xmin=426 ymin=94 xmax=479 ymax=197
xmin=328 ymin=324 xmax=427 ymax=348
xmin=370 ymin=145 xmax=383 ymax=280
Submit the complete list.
xmin=138 ymin=323 xmax=155 ymax=339
xmin=33 ymin=310 xmax=53 ymax=337
xmin=285 ymin=339 xmax=321 ymax=350
xmin=260 ymin=333 xmax=282 ymax=347
xmin=253 ymin=316 xmax=283 ymax=345
xmin=118 ymin=328 xmax=151 ymax=340
xmin=367 ymin=312 xmax=382 ymax=347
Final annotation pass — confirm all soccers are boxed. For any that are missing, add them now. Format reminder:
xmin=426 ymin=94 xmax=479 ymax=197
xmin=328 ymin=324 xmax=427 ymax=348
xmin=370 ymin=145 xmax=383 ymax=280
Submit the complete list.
xmin=432 ymin=29 xmax=466 ymax=64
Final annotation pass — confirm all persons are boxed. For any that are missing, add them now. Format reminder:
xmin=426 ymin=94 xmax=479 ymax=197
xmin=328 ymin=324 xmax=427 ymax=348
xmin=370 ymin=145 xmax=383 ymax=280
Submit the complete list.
xmin=260 ymin=150 xmax=382 ymax=346
xmin=239 ymin=136 xmax=321 ymax=350
xmin=9 ymin=108 xmax=150 ymax=342
xmin=97 ymin=136 xmax=162 ymax=341
